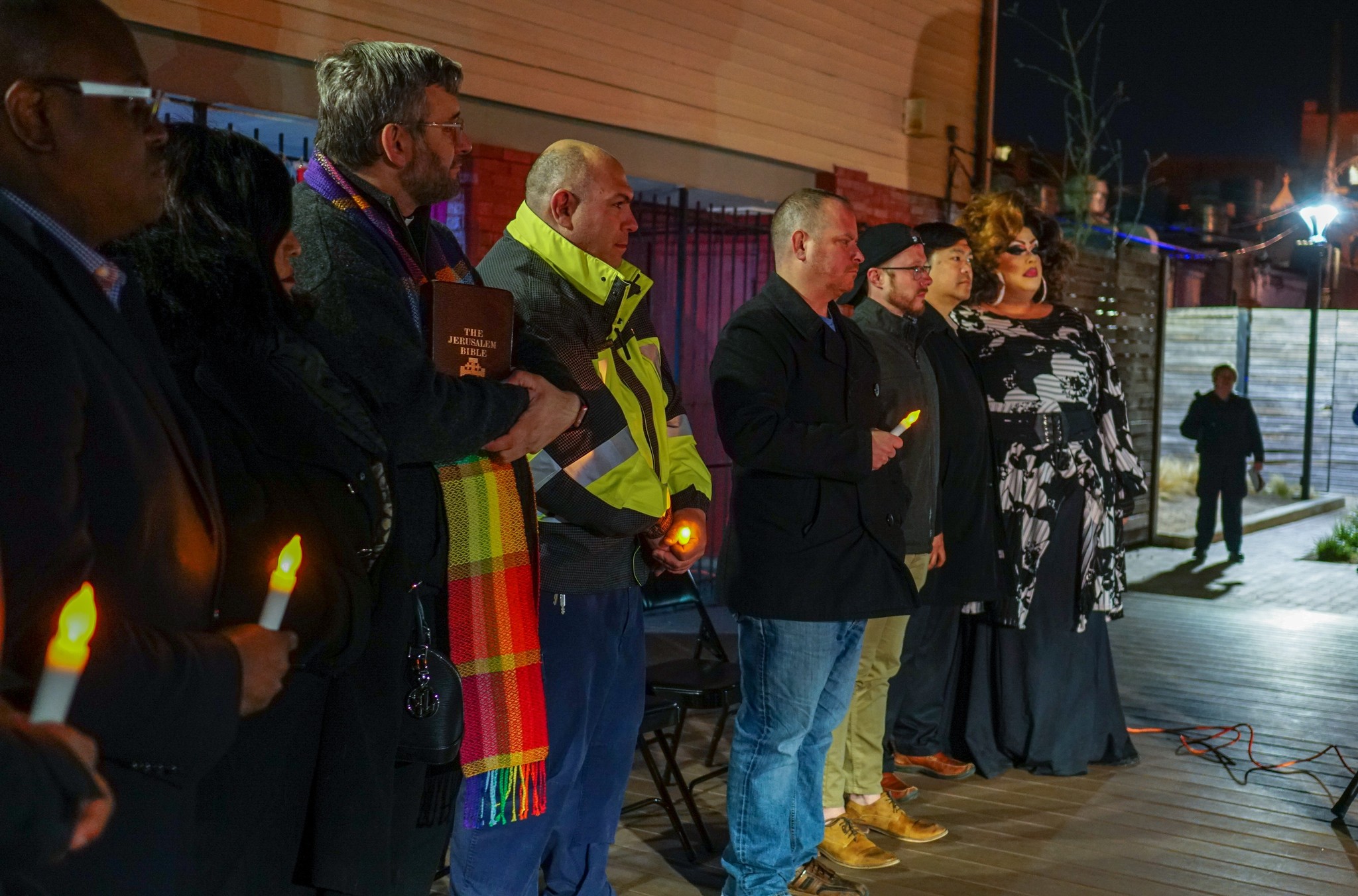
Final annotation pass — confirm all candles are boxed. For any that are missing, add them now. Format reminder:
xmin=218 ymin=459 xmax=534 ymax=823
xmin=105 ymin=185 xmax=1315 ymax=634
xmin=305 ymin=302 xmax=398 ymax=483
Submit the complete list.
xmin=676 ymin=526 xmax=692 ymax=546
xmin=892 ymin=406 xmax=922 ymax=439
xmin=261 ymin=531 xmax=301 ymax=631
xmin=31 ymin=579 xmax=97 ymax=732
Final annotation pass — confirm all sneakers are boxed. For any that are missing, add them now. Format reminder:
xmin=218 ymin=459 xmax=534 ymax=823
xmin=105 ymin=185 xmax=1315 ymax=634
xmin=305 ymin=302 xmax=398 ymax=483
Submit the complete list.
xmin=788 ymin=859 xmax=869 ymax=896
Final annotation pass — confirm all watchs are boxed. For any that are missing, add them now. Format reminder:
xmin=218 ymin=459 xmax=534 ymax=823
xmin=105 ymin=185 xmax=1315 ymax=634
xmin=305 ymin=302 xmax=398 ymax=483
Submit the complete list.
xmin=565 ymin=396 xmax=587 ymax=433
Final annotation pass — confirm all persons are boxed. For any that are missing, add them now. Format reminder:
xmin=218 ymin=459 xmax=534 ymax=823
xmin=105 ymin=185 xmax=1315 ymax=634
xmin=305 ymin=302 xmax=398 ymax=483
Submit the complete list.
xmin=449 ymin=137 xmax=712 ymax=896
xmin=1179 ymin=365 xmax=1263 ymax=563
xmin=820 ymin=191 xmax=1144 ymax=868
xmin=710 ymin=188 xmax=919 ymax=896
xmin=0 ymin=0 xmax=587 ymax=896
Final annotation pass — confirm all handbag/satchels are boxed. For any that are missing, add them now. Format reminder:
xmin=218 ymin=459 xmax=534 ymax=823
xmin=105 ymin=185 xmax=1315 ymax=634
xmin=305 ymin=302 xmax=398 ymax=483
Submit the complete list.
xmin=396 ymin=593 xmax=464 ymax=758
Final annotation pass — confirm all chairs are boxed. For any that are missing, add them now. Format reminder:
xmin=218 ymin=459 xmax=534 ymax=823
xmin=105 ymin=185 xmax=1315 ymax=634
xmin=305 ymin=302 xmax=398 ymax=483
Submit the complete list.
xmin=621 ymin=685 xmax=700 ymax=865
xmin=643 ymin=561 xmax=743 ymax=861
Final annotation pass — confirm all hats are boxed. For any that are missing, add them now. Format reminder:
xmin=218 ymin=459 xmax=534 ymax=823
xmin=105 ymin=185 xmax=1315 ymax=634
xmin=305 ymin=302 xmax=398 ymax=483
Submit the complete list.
xmin=835 ymin=221 xmax=925 ymax=306
xmin=915 ymin=221 xmax=969 ymax=259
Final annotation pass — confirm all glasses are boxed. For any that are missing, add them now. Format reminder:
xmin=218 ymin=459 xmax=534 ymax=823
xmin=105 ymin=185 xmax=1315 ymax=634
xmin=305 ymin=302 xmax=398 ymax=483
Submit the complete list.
xmin=402 ymin=117 xmax=467 ymax=145
xmin=26 ymin=75 xmax=163 ymax=133
xmin=880 ymin=265 xmax=932 ymax=281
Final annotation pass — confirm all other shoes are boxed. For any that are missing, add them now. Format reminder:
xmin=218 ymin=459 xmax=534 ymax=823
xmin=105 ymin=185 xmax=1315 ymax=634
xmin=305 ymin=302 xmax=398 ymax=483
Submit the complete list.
xmin=1194 ymin=547 xmax=1207 ymax=563
xmin=1229 ymin=551 xmax=1244 ymax=560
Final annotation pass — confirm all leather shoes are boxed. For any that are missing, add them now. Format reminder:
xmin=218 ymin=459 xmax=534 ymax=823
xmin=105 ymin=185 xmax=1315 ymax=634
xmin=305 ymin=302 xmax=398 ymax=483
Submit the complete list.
xmin=893 ymin=750 xmax=976 ymax=780
xmin=881 ymin=771 xmax=919 ymax=803
xmin=845 ymin=789 xmax=949 ymax=843
xmin=816 ymin=814 xmax=900 ymax=871
